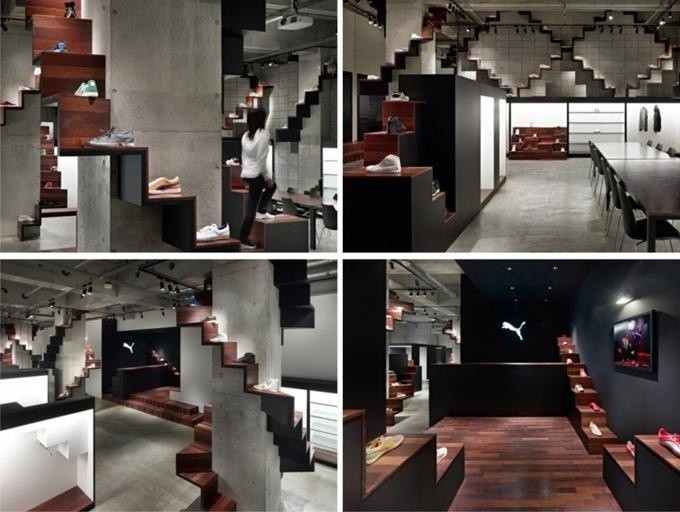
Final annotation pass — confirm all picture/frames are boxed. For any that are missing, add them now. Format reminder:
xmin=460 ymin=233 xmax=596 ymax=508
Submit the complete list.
xmin=612 ymin=308 xmax=657 ymax=373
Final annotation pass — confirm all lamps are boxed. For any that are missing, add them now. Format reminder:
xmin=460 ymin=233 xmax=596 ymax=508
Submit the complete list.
xmin=81 ymin=281 xmax=93 ymax=296
xmin=159 ymin=279 xmax=181 ymax=295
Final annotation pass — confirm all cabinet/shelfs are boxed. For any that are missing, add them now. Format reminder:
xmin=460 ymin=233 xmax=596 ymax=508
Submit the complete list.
xmin=567 ymin=102 xmax=626 ymax=158
xmin=505 ymin=127 xmax=568 ymax=160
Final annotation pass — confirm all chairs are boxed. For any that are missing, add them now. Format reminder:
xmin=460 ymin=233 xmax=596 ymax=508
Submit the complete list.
xmin=587 ymin=140 xmax=680 ymax=253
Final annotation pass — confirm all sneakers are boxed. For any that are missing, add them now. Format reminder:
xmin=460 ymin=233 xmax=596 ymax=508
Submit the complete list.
xmin=590 ymin=403 xmax=600 ymax=411
xmin=590 ymin=421 xmax=601 ymax=436
xmin=436 ymin=446 xmax=447 ymax=463
xmin=0 ymin=101 xmax=16 ymax=107
xmin=366 ymin=155 xmax=401 ymax=173
xmin=149 ymin=175 xmax=181 ymax=194
xmin=187 ymin=298 xmax=280 ymax=393
xmin=562 ymin=342 xmax=587 ymax=392
xmin=86 ymin=127 xmax=135 ymax=147
xmin=256 ymin=211 xmax=274 ymax=219
xmin=367 ymin=33 xmax=421 ymax=80
xmin=17 ymin=214 xmax=35 ymax=223
xmin=196 ymin=223 xmax=230 ymax=242
xmin=225 ymin=157 xmax=240 ymax=166
xmin=387 ymin=114 xmax=407 ymax=132
xmin=659 ymin=428 xmax=680 ymax=457
xmin=627 ymin=441 xmax=636 ymax=458
xmin=366 ymin=435 xmax=404 ymax=465
xmin=390 ymin=91 xmax=409 ymax=102
xmin=19 ymin=85 xmax=34 ymax=90
xmin=74 ymin=79 xmax=98 ymax=97
xmin=240 ymin=239 xmax=257 ymax=249
xmin=40 ymin=135 xmax=57 ymax=188
xmin=397 ymin=392 xmax=407 ymax=398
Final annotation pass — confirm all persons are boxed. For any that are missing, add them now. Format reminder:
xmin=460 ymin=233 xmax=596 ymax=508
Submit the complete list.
xmin=238 ymin=107 xmax=277 ymax=250
